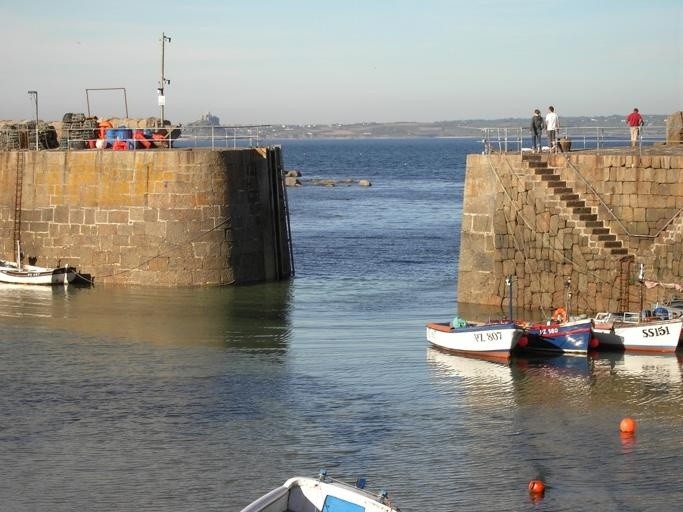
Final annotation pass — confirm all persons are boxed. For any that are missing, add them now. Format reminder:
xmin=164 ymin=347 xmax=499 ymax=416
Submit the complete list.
xmin=528 ymin=107 xmax=545 ymax=152
xmin=546 ymin=104 xmax=560 ymax=150
xmin=623 ymin=109 xmax=645 ymax=146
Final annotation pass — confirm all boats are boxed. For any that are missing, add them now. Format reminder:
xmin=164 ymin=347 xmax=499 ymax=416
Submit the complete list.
xmin=426 ymin=347 xmax=682 ymax=395
xmin=423 ymin=261 xmax=683 ymax=359
xmin=240 ymin=469 xmax=400 ymax=511
xmin=0 ymin=238 xmax=75 ymax=286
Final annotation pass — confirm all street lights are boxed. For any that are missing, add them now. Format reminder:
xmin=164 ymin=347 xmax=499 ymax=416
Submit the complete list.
xmin=157 ymin=32 xmax=171 ymax=127
xmin=28 ymin=90 xmax=39 ymax=151
